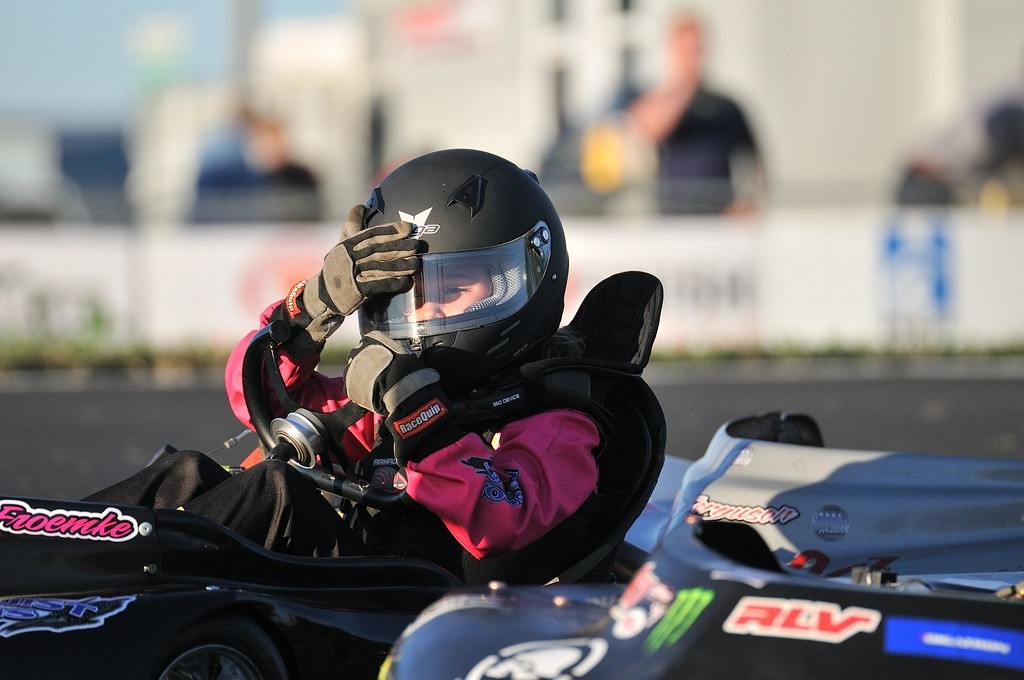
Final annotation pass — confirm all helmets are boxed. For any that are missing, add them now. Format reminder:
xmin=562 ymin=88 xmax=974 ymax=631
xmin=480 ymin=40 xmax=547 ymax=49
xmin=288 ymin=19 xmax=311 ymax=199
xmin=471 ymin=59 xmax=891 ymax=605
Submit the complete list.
xmin=357 ymin=148 xmax=570 ymax=400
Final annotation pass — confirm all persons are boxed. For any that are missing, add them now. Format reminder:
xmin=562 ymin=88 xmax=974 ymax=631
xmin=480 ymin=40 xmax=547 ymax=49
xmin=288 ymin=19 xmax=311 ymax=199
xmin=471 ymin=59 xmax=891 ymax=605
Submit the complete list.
xmin=634 ymin=18 xmax=762 ymax=215
xmin=78 ymin=148 xmax=615 ymax=586
xmin=192 ymin=114 xmax=322 ymax=222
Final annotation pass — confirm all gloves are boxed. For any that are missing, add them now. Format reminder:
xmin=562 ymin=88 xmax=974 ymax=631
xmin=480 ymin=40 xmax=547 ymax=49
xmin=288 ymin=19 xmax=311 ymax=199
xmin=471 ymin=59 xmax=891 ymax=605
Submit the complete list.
xmin=342 ymin=331 xmax=441 ymax=421
xmin=280 ymin=204 xmax=420 ymax=359
